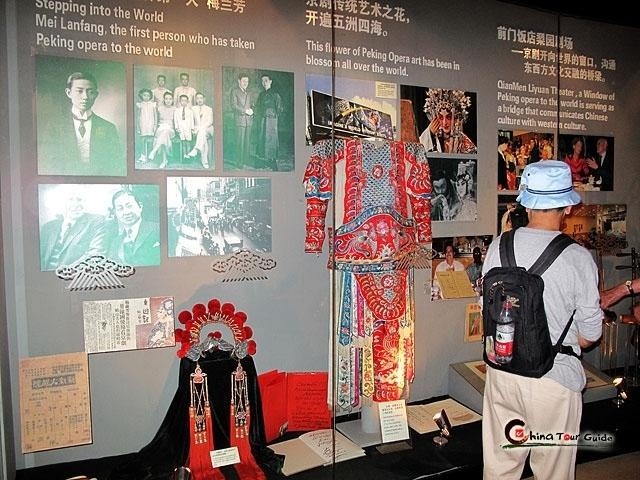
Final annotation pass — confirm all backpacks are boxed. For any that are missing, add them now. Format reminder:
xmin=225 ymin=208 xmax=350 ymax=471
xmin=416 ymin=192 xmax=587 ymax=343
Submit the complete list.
xmin=482 ymin=226 xmax=583 ymax=378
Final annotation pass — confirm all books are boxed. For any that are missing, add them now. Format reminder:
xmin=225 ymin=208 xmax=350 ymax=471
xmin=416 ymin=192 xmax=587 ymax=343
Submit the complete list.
xmin=265 ymin=428 xmax=366 ymax=476
xmin=406 ymin=398 xmax=483 ymax=435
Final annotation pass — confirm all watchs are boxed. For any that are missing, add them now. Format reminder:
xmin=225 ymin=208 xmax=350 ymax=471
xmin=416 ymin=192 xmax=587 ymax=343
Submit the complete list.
xmin=625 ymin=280 xmax=634 ymax=295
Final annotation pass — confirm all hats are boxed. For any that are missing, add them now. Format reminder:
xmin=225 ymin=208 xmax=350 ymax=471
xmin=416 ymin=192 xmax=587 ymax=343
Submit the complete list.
xmin=520 ymin=160 xmax=582 ymax=210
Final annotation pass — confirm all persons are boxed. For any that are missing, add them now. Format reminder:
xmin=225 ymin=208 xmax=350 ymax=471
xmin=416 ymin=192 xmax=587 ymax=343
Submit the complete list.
xmin=628 ymin=301 xmax=640 ymax=378
xmin=481 ymin=160 xmax=602 ymax=480
xmin=599 ymin=278 xmax=640 ymax=324
xmin=40 ymin=73 xmax=282 ymax=271
xmin=329 ymin=88 xmax=613 ymax=301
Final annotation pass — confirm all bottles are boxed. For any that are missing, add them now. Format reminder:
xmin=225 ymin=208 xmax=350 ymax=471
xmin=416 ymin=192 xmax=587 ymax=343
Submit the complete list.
xmin=494 ymin=300 xmax=516 ymax=363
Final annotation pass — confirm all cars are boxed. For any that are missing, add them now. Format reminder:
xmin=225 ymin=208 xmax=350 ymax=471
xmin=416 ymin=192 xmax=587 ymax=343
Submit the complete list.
xmin=222 ymin=237 xmax=245 ymax=255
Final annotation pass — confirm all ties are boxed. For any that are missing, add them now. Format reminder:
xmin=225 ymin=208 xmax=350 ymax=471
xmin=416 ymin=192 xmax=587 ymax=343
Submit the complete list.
xmin=182 ymin=106 xmax=185 ymax=120
xmin=72 ymin=113 xmax=93 ymax=138
xmin=62 ymin=223 xmax=71 ymax=244
